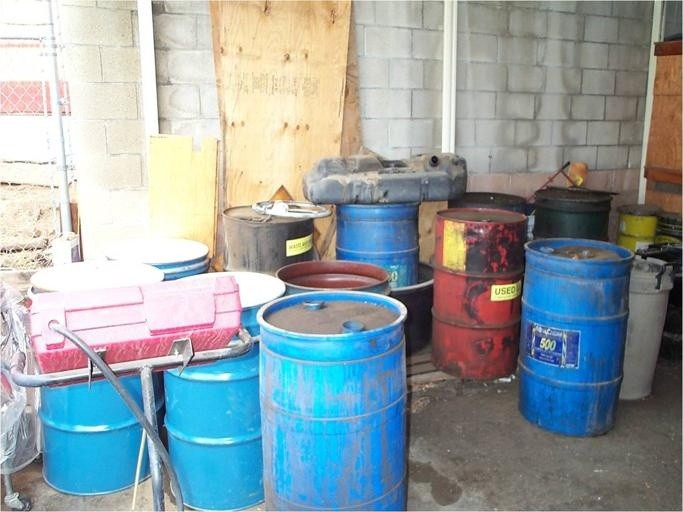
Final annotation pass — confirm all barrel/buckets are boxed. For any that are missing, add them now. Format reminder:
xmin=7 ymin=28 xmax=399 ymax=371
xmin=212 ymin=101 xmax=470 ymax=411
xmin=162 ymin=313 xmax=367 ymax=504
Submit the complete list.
xmin=447 ymin=192 xmax=526 ymax=215
xmin=617 ymin=203 xmax=659 ymax=252
xmin=616 ymin=252 xmax=675 ymax=401
xmin=29 ymin=260 xmax=164 ymax=497
xmin=430 ymin=208 xmax=526 ymax=382
xmin=108 ymin=239 xmax=210 ymax=281
xmin=334 ymin=200 xmax=421 ymax=287
xmin=254 ymin=289 xmax=408 ymax=512
xmin=384 ymin=262 xmax=434 ymax=353
xmin=222 ymin=205 xmax=315 ymax=276
xmin=275 ymin=260 xmax=391 ymax=299
xmin=652 ymin=212 xmax=682 ymax=364
xmin=158 ymin=268 xmax=288 ymax=512
xmin=532 ymin=188 xmax=612 ymax=243
